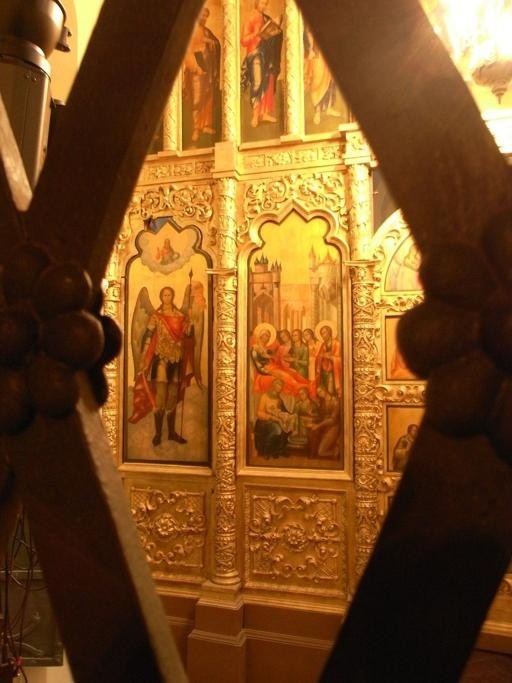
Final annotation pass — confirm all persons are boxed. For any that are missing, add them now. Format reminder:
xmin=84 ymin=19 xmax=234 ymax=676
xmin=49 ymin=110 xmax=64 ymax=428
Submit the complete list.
xmin=182 ymin=7 xmax=219 ymax=142
xmin=240 ymin=0 xmax=285 ymax=128
xmin=248 ymin=327 xmax=343 ymax=459
xmin=137 ymin=287 xmax=194 ymax=447
xmin=395 ymin=422 xmax=418 ymax=470
xmin=154 ymin=236 xmax=179 ymax=266
xmin=306 ymin=25 xmax=341 ymax=126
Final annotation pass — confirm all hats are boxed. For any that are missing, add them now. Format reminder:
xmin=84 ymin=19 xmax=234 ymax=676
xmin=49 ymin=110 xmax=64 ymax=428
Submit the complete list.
xmin=314 ymin=319 xmax=338 ymax=343
xmin=253 ymin=322 xmax=276 ymax=348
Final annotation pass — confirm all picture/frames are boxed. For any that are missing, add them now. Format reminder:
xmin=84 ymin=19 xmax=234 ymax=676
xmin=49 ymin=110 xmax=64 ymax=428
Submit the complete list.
xmin=381 ymin=397 xmax=431 ymax=475
xmin=236 ymin=195 xmax=359 ymax=486
xmin=238 ymin=1 xmax=292 ymax=150
xmin=299 ymin=5 xmax=354 ymax=144
xmin=177 ymin=0 xmax=228 ymax=155
xmin=377 ymin=314 xmax=427 ymax=385
xmin=112 ymin=217 xmax=216 ymax=477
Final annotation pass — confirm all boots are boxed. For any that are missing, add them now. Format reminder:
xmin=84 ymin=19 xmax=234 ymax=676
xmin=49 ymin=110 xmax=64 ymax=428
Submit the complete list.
xmin=152 ymin=407 xmax=165 ymax=445
xmin=165 ymin=408 xmax=187 ymax=443
